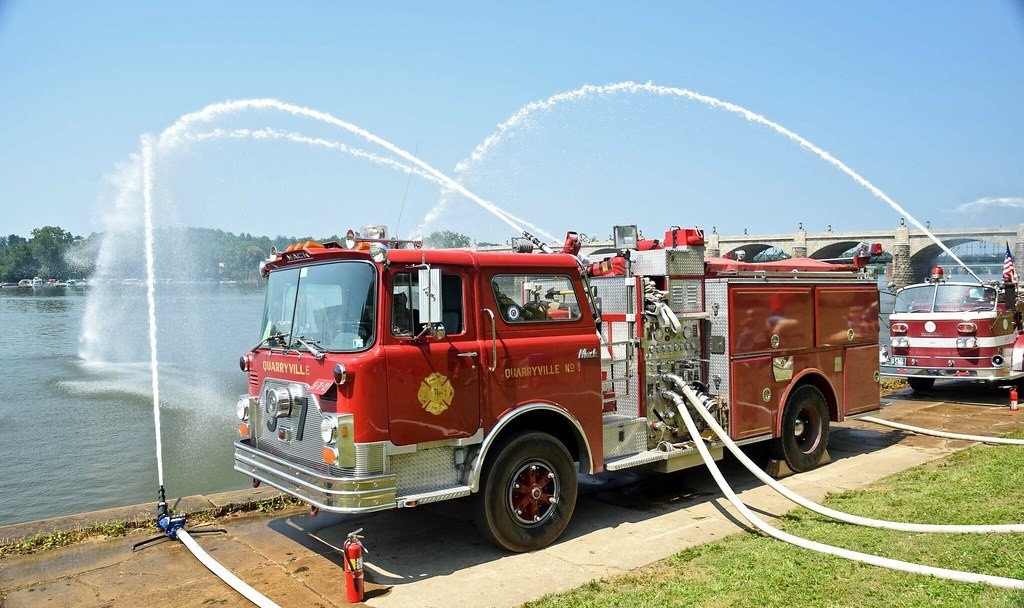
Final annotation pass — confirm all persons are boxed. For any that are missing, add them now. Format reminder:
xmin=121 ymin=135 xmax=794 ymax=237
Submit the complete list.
xmin=736 ymin=305 xmax=799 ymax=351
xmin=843 ymin=305 xmax=878 ymax=332
xmin=493 ymin=282 xmax=536 ymax=321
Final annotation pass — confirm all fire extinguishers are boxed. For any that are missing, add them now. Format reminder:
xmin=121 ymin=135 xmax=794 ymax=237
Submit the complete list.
xmin=1009 ymin=385 xmax=1019 ymax=411
xmin=342 ymin=527 xmax=368 ymax=604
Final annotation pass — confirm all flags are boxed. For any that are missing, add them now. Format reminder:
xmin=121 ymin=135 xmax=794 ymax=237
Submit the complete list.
xmin=1003 ymin=246 xmax=1019 ymax=285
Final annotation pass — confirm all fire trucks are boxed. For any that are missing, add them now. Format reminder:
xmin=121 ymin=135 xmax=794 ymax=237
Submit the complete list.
xmin=232 ymin=226 xmax=894 ymax=556
xmin=879 ymin=263 xmax=1024 ymax=388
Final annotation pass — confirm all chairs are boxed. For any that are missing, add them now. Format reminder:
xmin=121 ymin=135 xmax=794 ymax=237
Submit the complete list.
xmin=393 ymin=292 xmax=410 ymax=332
xmin=427 ymin=313 xmax=460 ymax=334
